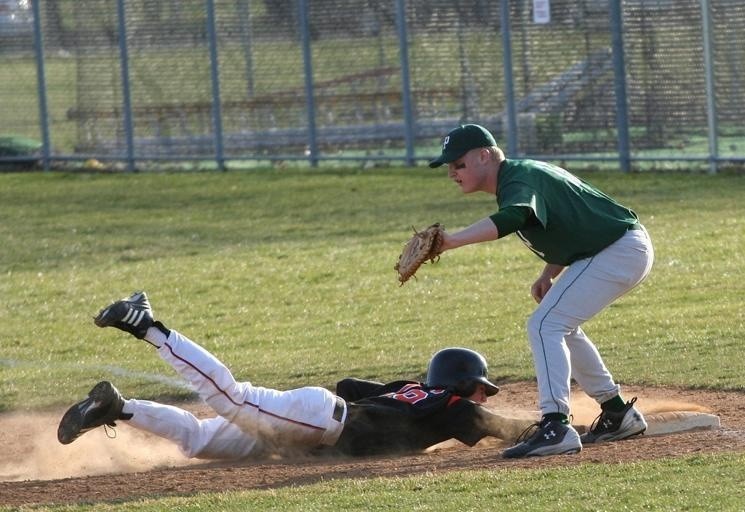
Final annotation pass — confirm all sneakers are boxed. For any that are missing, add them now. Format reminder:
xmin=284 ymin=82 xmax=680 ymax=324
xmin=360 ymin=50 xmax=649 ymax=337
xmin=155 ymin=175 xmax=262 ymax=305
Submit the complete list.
xmin=57 ymin=380 xmax=125 ymax=444
xmin=93 ymin=291 xmax=154 ymax=339
xmin=503 ymin=397 xmax=648 ymax=458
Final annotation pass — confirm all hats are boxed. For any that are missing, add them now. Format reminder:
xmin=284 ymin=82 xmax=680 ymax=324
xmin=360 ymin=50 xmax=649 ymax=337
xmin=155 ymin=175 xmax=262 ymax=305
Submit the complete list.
xmin=429 ymin=124 xmax=497 ymax=168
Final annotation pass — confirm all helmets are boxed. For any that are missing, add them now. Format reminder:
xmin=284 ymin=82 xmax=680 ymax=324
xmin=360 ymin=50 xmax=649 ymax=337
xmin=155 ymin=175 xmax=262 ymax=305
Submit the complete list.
xmin=426 ymin=347 xmax=499 ymax=396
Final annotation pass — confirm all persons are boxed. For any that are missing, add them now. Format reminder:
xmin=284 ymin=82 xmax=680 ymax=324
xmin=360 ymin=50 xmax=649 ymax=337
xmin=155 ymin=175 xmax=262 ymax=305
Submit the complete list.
xmin=394 ymin=123 xmax=654 ymax=459
xmin=57 ymin=290 xmax=597 ymax=461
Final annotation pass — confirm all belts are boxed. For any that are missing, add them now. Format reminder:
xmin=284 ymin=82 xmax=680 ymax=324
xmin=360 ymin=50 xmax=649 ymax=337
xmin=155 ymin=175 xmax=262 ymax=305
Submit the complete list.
xmin=628 ymin=224 xmax=641 ymax=230
xmin=315 ymin=396 xmax=344 ymax=453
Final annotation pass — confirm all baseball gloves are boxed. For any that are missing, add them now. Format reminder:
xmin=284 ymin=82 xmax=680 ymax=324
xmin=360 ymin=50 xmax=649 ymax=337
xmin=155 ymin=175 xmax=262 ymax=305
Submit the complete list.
xmin=396 ymin=222 xmax=444 ymax=282
